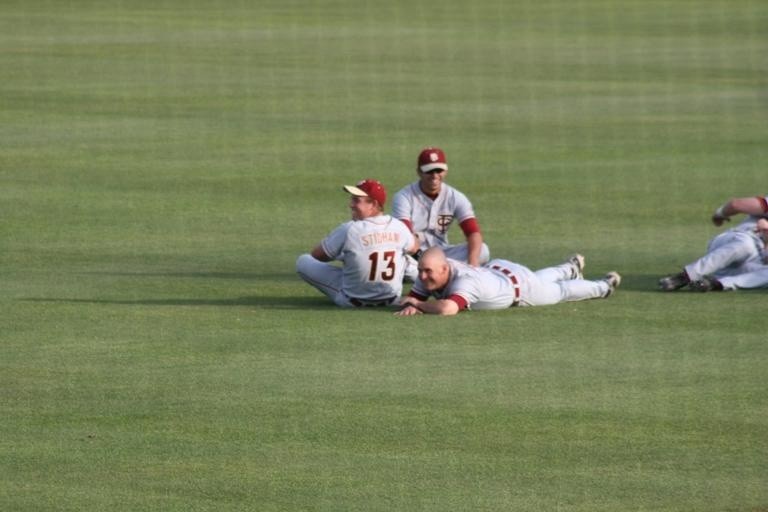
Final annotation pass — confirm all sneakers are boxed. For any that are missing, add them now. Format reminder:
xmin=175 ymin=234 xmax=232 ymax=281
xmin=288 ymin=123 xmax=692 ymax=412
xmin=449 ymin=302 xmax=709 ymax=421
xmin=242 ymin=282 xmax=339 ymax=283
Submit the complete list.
xmin=606 ymin=271 xmax=621 ymax=288
xmin=659 ymin=272 xmax=688 ymax=292
xmin=573 ymin=254 xmax=585 ymax=271
xmin=689 ymin=274 xmax=716 ymax=292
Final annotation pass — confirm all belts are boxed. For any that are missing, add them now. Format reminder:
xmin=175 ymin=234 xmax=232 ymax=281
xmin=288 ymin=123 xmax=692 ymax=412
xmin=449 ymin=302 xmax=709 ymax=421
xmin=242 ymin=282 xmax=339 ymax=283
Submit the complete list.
xmin=345 ymin=296 xmax=397 ymax=307
xmin=493 ymin=265 xmax=519 ymax=306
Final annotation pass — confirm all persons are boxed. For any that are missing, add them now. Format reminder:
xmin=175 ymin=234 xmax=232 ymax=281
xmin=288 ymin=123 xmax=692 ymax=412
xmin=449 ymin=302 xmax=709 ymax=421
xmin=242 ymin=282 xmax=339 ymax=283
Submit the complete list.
xmin=390 ymin=143 xmax=490 ymax=285
xmin=295 ymin=178 xmax=420 ymax=309
xmin=391 ymin=244 xmax=622 ymax=318
xmin=654 ymin=193 xmax=767 ymax=293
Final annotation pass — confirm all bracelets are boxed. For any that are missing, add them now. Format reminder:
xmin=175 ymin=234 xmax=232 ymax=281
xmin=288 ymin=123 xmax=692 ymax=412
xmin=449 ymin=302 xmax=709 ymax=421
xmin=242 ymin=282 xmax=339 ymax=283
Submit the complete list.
xmin=715 ymin=206 xmax=727 ymax=221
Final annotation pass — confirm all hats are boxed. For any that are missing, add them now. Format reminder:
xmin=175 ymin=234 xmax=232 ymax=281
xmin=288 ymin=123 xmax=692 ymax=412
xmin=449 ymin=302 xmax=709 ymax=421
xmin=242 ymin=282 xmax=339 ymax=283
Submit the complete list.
xmin=344 ymin=179 xmax=387 ymax=207
xmin=419 ymin=147 xmax=448 ymax=173
